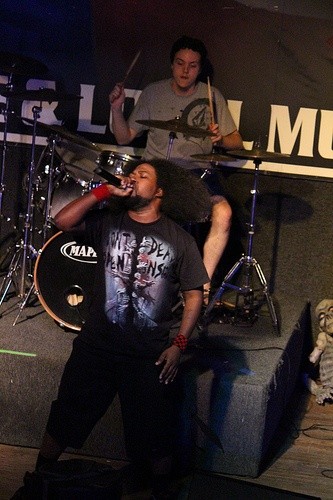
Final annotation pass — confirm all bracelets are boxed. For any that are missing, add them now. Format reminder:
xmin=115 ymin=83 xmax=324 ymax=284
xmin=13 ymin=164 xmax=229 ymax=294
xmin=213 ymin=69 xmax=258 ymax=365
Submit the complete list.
xmin=173 ymin=335 xmax=187 ymax=351
xmin=91 ymin=183 xmax=109 ymax=201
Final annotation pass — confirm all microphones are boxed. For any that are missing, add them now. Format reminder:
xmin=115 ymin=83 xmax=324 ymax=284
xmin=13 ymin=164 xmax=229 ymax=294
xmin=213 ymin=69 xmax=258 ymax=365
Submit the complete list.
xmin=92 ymin=166 xmax=127 ymax=191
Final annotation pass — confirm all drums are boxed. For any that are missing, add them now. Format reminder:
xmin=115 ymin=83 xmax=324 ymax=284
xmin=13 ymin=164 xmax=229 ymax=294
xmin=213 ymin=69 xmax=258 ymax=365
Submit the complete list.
xmin=37 ymin=161 xmax=110 ymax=226
xmin=19 ymin=138 xmax=101 ymax=214
xmin=93 ymin=150 xmax=145 ymax=181
xmin=33 ymin=226 xmax=107 ymax=332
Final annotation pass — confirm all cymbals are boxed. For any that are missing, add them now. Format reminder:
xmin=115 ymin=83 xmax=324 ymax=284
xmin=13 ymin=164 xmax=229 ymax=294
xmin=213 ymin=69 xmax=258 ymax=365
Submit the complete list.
xmin=18 ymin=116 xmax=103 ymax=153
xmin=134 ymin=118 xmax=219 ymax=138
xmin=228 ymin=148 xmax=292 ymax=163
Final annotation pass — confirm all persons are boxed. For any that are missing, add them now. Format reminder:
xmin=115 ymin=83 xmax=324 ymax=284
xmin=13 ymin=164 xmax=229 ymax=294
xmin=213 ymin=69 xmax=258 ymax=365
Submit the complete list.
xmin=109 ymin=36 xmax=243 ymax=306
xmin=306 ymin=298 xmax=333 ymax=404
xmin=35 ymin=159 xmax=212 ymax=500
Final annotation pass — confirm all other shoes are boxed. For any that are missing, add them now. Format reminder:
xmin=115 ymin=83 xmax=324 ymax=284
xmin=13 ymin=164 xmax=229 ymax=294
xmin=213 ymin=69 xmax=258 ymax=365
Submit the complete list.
xmin=200 ymin=307 xmax=252 ymax=327
xmin=12 ymin=472 xmax=50 ymax=500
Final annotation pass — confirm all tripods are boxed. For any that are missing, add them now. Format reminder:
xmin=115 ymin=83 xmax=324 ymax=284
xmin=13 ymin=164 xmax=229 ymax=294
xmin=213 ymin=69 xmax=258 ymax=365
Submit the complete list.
xmin=201 ymin=158 xmax=282 ymax=337
xmin=0 ymin=94 xmax=60 ymax=325
